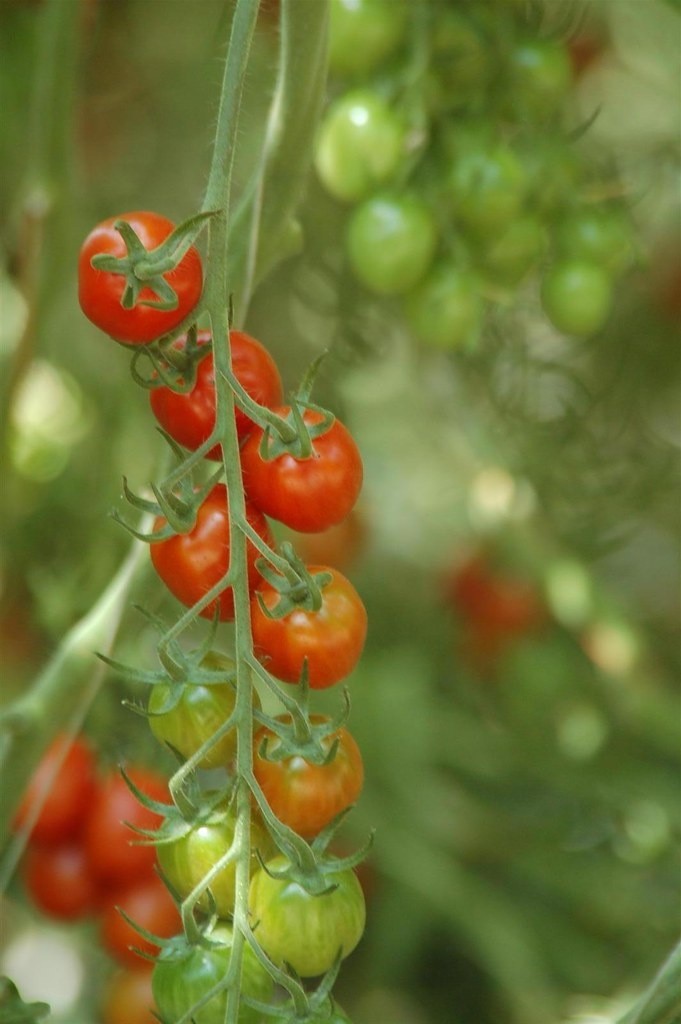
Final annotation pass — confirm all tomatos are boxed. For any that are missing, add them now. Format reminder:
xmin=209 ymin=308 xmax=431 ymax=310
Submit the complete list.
xmin=0 ymin=0 xmax=681 ymax=1024
